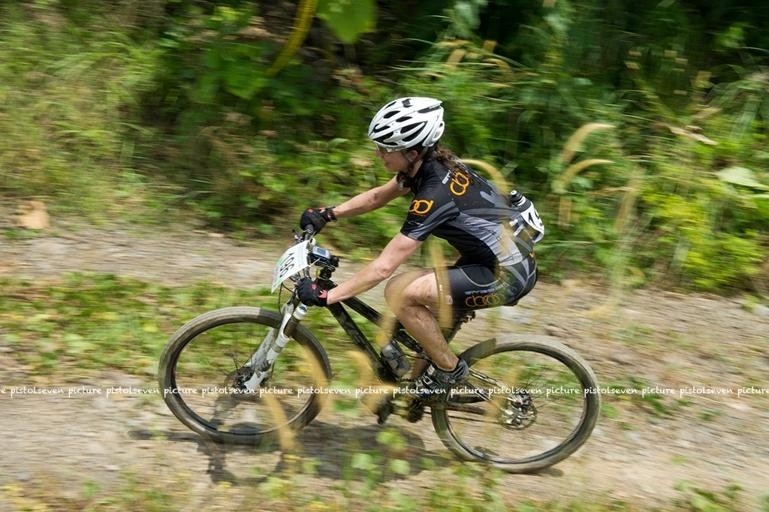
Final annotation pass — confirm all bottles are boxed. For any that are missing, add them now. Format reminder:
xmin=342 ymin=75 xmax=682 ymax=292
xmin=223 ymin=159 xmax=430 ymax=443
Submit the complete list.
xmin=507 ymin=189 xmax=541 ymax=240
xmin=376 ymin=334 xmax=412 ymax=378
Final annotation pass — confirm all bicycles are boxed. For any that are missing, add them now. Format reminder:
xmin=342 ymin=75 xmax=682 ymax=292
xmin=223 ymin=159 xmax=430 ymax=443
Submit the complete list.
xmin=157 ymin=221 xmax=601 ymax=473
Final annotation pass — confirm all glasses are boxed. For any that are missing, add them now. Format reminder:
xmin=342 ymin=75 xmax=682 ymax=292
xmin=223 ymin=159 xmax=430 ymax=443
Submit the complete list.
xmin=377 ymin=144 xmax=405 ymax=153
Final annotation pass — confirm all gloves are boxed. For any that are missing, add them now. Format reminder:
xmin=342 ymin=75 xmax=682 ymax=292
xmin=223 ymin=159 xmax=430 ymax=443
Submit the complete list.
xmin=296 ymin=278 xmax=329 ymax=307
xmin=301 ymin=206 xmax=337 ymax=231
xmin=407 ymin=356 xmax=469 ymax=399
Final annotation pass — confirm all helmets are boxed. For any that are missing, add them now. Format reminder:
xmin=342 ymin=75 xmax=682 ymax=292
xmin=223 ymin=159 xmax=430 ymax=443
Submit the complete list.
xmin=369 ymin=97 xmax=444 ymax=152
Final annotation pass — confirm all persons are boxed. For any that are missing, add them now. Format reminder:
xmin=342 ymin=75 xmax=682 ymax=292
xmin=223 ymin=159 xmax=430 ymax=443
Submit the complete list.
xmin=293 ymin=95 xmax=544 ymax=424
